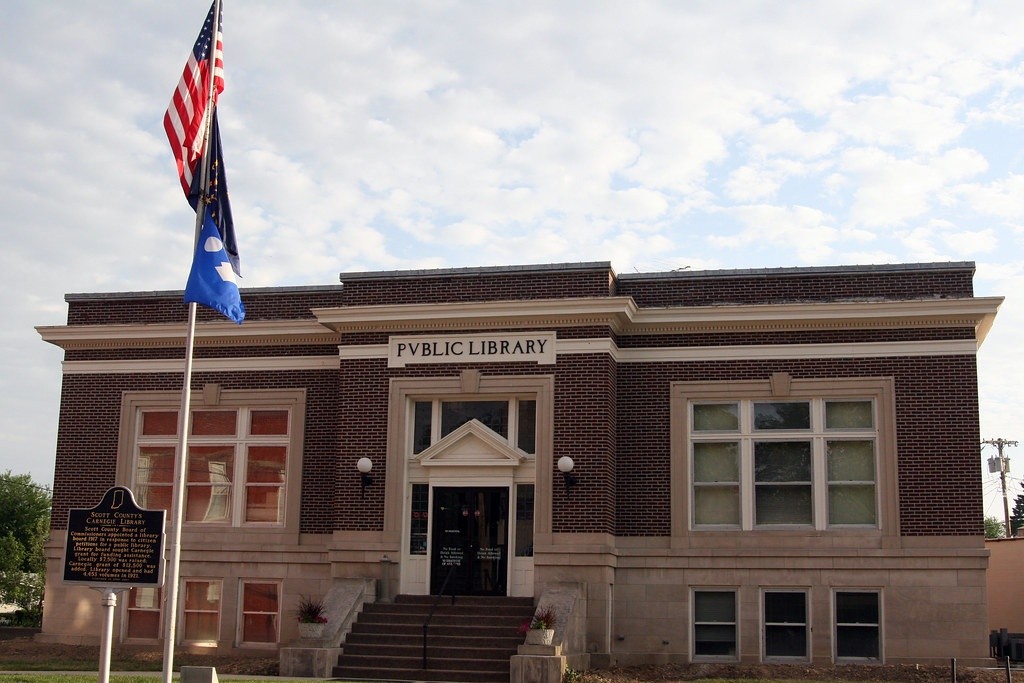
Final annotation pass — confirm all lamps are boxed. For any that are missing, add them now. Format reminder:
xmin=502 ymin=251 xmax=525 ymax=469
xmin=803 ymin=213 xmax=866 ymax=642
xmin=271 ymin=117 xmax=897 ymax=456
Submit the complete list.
xmin=357 ymin=458 xmax=374 ymax=487
xmin=558 ymin=456 xmax=577 ymax=488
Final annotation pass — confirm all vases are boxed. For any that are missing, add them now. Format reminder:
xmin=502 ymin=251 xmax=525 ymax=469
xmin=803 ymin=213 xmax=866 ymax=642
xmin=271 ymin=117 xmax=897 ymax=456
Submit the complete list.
xmin=526 ymin=628 xmax=555 ymax=646
xmin=297 ymin=623 xmax=325 ymax=640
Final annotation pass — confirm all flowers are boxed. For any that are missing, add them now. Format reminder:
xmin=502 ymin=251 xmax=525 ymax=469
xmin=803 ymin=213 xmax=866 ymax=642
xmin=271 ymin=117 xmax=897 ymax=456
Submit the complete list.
xmin=287 ymin=592 xmax=328 ymax=625
xmin=518 ymin=604 xmax=559 ymax=635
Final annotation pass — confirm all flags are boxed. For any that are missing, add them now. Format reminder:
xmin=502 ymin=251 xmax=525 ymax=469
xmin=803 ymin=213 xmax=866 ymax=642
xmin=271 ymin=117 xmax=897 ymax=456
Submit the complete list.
xmin=184 ymin=107 xmax=244 ymax=278
xmin=164 ymin=0 xmax=225 ymax=197
xmin=182 ymin=205 xmax=247 ymax=326
